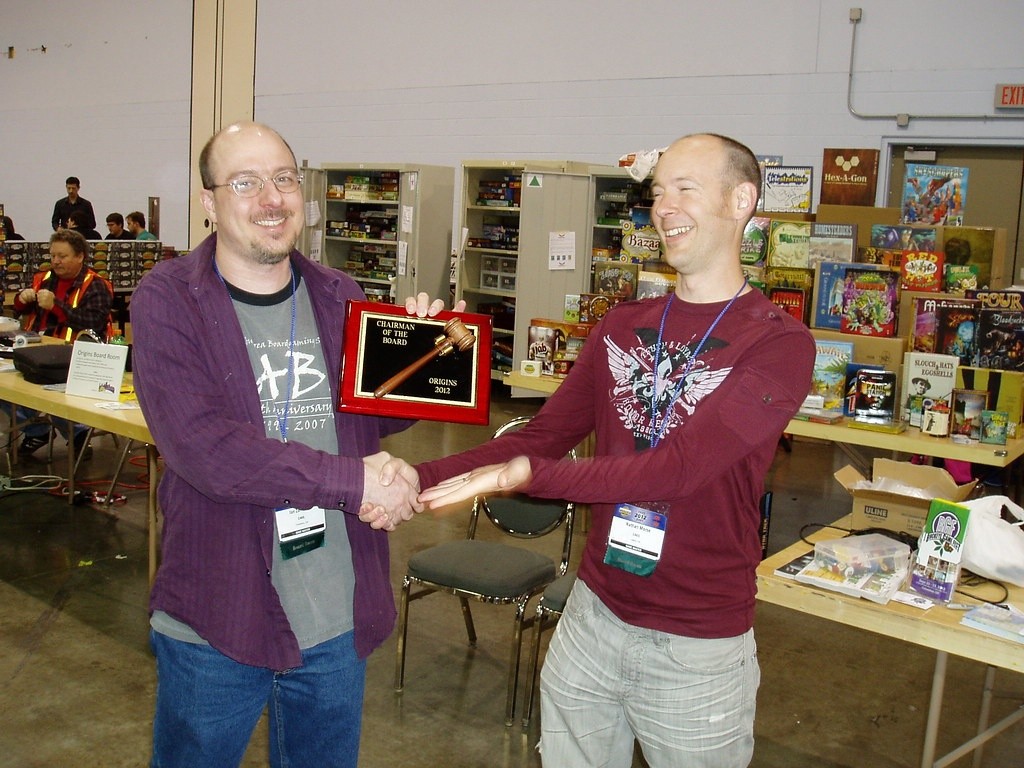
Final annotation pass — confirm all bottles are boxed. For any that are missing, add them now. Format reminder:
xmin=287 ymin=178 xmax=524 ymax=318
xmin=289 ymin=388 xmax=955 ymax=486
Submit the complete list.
xmin=110 ymin=330 xmax=128 ymax=377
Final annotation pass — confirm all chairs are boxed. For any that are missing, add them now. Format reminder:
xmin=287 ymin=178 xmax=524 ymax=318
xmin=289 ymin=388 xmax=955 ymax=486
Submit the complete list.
xmin=394 ymin=417 xmax=578 ymax=727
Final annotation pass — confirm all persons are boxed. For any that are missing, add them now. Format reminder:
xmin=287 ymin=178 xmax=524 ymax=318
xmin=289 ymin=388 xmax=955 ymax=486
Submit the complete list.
xmin=128 ymin=122 xmax=468 ymax=768
xmin=0 ymin=176 xmax=156 ymax=459
xmin=355 ymin=134 xmax=818 ymax=768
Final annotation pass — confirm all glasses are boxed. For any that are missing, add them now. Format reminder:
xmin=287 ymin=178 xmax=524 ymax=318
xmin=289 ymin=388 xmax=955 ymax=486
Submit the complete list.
xmin=207 ymin=170 xmax=300 ymax=200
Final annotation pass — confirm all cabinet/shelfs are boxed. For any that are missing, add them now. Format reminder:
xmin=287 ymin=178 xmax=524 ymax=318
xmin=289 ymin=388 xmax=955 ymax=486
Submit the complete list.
xmin=301 ymin=164 xmax=455 ymax=321
xmin=459 ymin=160 xmax=664 ymax=431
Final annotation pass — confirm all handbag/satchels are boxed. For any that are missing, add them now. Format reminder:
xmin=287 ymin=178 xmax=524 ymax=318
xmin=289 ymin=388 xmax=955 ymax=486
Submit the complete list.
xmin=13 ymin=344 xmax=73 ymax=385
xmin=918 ymin=495 xmax=1024 ymax=588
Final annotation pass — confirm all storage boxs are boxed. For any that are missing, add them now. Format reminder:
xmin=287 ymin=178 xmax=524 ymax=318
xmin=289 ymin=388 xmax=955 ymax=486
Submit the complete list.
xmin=526 ymin=148 xmax=1024 ymax=445
xmin=835 ymin=456 xmax=979 ymax=541
xmin=816 ymin=534 xmax=911 ymax=576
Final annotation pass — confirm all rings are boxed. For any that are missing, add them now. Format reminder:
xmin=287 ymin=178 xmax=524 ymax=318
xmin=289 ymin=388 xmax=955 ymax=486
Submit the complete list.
xmin=463 ymin=478 xmax=466 ymax=482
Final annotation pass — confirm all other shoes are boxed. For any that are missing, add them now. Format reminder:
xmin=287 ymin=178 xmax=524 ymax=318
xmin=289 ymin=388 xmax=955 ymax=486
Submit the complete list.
xmin=73 ymin=429 xmax=93 ymax=460
xmin=18 ymin=428 xmax=57 ymax=455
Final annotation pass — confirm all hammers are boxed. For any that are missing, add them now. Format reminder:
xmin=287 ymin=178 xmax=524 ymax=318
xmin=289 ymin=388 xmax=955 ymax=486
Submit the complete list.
xmin=376 ymin=317 xmax=475 ymax=395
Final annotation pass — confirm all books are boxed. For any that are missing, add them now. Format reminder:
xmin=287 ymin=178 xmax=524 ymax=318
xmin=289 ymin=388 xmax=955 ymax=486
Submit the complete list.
xmin=526 ymin=148 xmax=1024 ymax=644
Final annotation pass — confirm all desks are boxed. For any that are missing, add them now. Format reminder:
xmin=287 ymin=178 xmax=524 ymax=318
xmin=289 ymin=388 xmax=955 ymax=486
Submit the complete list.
xmin=501 ymin=369 xmax=1024 ymax=767
xmin=0 ymin=336 xmax=161 ymax=614
